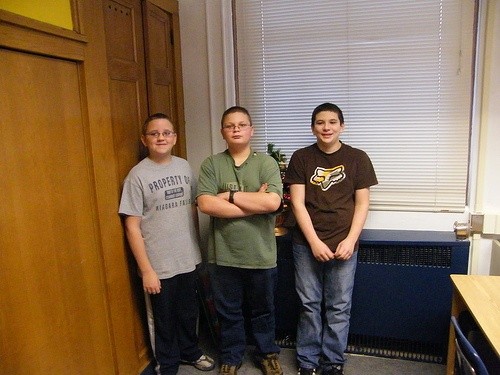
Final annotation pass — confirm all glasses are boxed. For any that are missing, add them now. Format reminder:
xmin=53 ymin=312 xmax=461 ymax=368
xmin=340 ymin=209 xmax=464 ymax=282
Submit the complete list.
xmin=221 ymin=122 xmax=252 ymax=128
xmin=144 ymin=130 xmax=176 ymax=137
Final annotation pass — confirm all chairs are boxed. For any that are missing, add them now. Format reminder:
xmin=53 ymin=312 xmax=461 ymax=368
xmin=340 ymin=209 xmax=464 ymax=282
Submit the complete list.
xmin=451 ymin=316 xmax=489 ymax=375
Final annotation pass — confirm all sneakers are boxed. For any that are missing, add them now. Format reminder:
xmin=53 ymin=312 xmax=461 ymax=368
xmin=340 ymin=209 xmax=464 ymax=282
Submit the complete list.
xmin=218 ymin=363 xmax=239 ymax=375
xmin=322 ymin=365 xmax=344 ymax=375
xmin=296 ymin=366 xmax=316 ymax=375
xmin=256 ymin=352 xmax=283 ymax=375
xmin=177 ymin=353 xmax=215 ymax=371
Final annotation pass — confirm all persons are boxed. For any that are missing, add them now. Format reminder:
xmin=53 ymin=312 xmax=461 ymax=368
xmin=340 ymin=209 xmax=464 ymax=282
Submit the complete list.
xmin=118 ymin=112 xmax=216 ymax=375
xmin=196 ymin=106 xmax=283 ymax=375
xmin=285 ymin=102 xmax=378 ymax=375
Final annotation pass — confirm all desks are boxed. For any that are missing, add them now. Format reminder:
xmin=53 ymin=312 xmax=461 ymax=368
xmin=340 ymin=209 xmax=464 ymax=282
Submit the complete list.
xmin=446 ymin=274 xmax=500 ymax=375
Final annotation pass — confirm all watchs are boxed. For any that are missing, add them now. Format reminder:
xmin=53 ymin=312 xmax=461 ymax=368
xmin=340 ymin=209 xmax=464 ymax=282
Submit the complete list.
xmin=228 ymin=189 xmax=238 ymax=203
